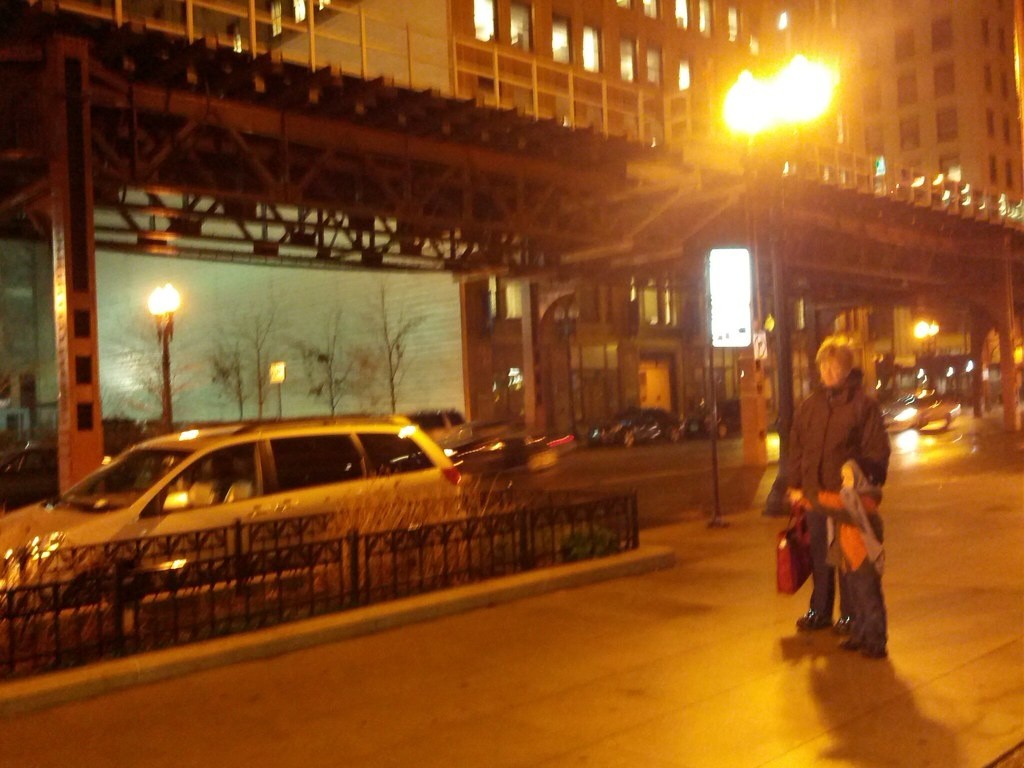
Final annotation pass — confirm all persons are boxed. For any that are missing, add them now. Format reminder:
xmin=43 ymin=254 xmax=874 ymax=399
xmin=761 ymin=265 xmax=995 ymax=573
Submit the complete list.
xmin=784 ymin=334 xmax=891 ymax=656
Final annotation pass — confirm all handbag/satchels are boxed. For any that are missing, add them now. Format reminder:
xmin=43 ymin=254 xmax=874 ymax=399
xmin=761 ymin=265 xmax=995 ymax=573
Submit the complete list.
xmin=774 ymin=499 xmax=815 ymax=596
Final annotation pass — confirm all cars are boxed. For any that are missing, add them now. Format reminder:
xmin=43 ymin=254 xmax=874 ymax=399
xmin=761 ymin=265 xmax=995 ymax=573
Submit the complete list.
xmin=0 ymin=409 xmax=464 ymax=626
xmin=408 ymin=374 xmax=965 ymax=477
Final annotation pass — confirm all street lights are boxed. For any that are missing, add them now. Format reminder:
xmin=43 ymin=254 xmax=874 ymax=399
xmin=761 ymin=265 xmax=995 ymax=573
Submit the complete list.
xmin=723 ymin=46 xmax=844 ymax=454
xmin=147 ymin=284 xmax=183 ymax=434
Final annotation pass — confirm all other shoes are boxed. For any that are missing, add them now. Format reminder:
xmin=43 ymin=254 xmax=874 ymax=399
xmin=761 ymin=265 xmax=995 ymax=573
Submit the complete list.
xmin=796 ymin=608 xmax=833 ymax=632
xmin=859 ymin=644 xmax=888 ymax=659
xmin=837 ymin=638 xmax=862 ymax=651
xmin=831 ymin=614 xmax=856 ymax=634
